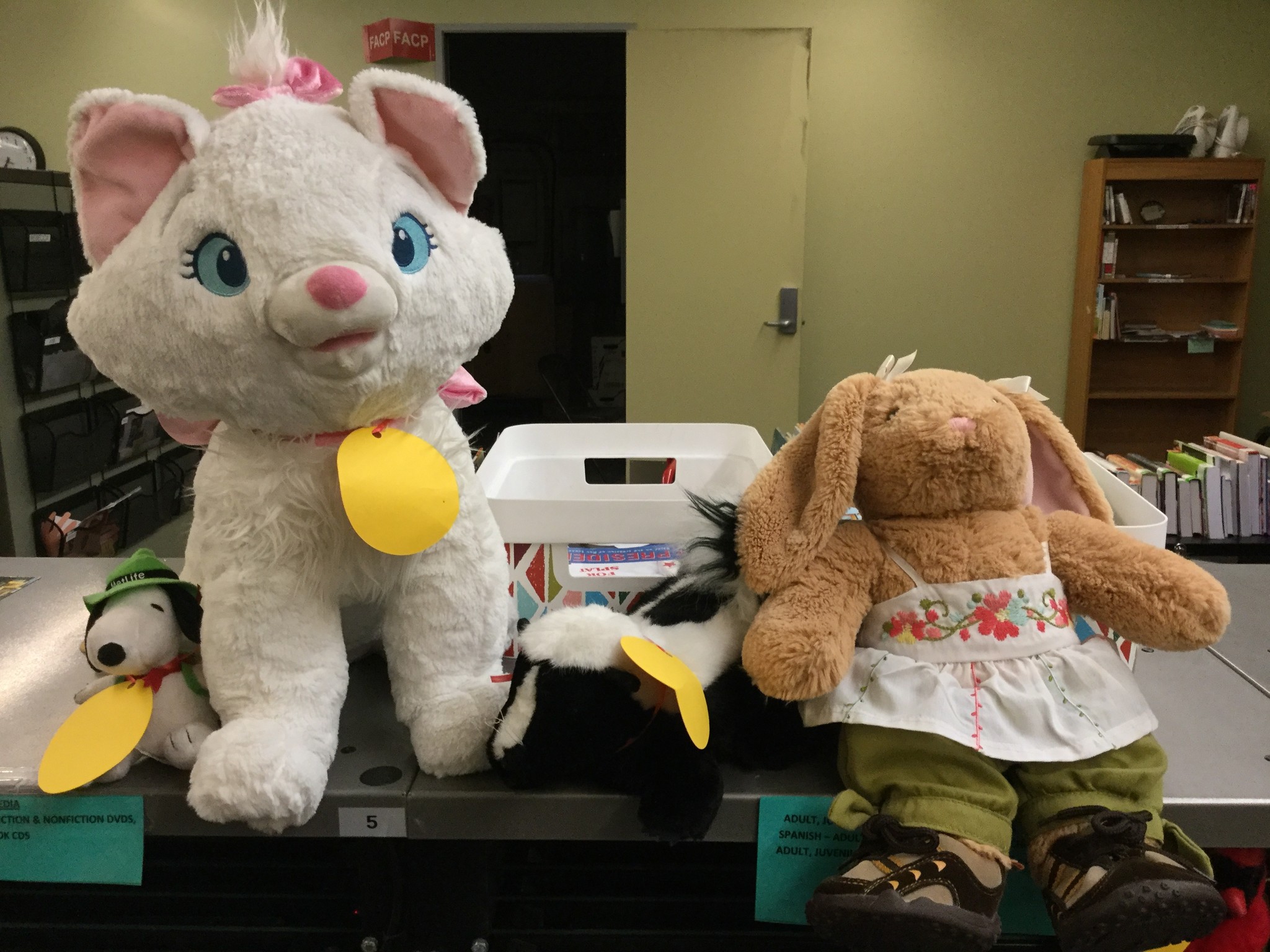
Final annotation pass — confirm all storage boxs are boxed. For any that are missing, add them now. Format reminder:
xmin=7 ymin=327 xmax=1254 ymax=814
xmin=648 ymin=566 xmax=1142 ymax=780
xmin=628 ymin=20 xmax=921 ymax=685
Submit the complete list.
xmin=476 ymin=422 xmax=775 ymax=684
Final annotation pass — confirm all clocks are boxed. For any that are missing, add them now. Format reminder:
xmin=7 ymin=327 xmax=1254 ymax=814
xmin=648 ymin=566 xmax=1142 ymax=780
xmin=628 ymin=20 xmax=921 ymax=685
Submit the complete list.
xmin=0 ymin=125 xmax=48 ymax=171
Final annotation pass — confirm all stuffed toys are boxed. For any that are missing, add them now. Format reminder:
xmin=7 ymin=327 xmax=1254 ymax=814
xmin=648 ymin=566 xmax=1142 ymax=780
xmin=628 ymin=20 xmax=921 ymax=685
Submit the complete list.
xmin=736 ymin=351 xmax=1231 ymax=952
xmin=485 ymin=491 xmax=796 ymax=840
xmin=73 ymin=548 xmax=221 ymax=783
xmin=66 ymin=0 xmax=514 ymax=833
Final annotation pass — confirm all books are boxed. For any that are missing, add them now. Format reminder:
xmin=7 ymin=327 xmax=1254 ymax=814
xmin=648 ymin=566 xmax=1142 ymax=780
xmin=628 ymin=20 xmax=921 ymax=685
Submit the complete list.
xmin=1104 ymin=186 xmax=1131 ymax=224
xmin=1084 ymin=432 xmax=1270 ymax=539
xmin=1101 ymin=232 xmax=1118 ymax=278
xmin=1227 ymin=183 xmax=1256 ymax=224
xmin=1093 ymin=284 xmax=1121 ymax=340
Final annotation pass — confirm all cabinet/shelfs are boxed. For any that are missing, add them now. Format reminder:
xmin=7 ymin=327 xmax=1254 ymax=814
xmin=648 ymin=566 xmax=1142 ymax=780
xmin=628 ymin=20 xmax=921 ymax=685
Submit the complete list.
xmin=0 ymin=168 xmax=197 ymax=557
xmin=1063 ymin=157 xmax=1265 ymax=463
